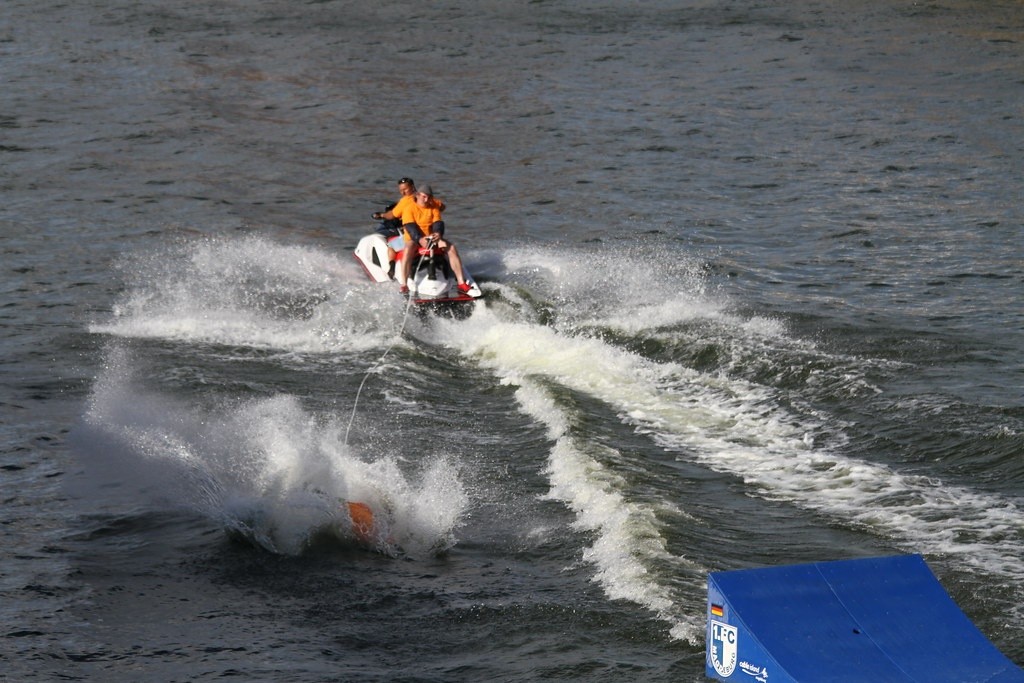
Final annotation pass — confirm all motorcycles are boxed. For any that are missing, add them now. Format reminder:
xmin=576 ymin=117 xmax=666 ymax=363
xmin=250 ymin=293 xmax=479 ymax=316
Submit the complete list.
xmin=352 ymin=202 xmax=481 ymax=305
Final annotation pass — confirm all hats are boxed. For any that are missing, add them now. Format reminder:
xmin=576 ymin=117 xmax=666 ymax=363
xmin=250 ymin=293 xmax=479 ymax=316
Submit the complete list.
xmin=419 ymin=184 xmax=435 ymax=198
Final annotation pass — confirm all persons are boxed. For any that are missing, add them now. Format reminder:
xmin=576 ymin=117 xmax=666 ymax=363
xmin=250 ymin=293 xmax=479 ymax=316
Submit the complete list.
xmin=371 ymin=178 xmax=471 ymax=294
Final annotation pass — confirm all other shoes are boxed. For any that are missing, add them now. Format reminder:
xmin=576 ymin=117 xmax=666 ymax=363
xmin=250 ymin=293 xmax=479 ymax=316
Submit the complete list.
xmin=456 ymin=282 xmax=472 ymax=292
xmin=400 ymin=285 xmax=410 ymax=293
